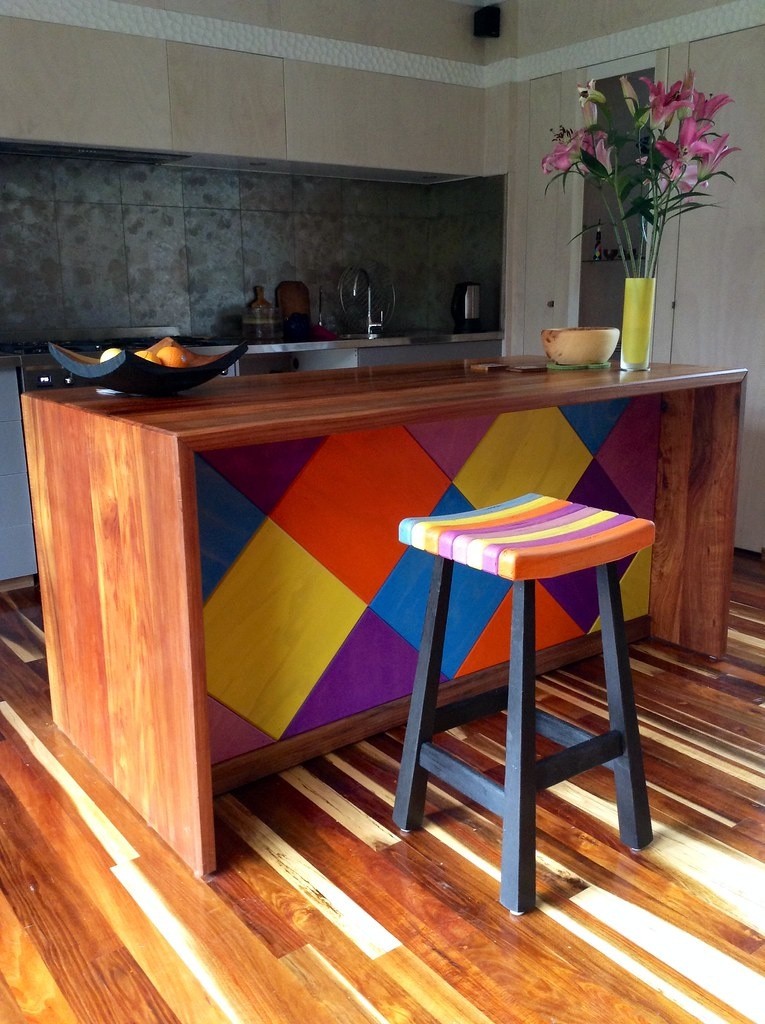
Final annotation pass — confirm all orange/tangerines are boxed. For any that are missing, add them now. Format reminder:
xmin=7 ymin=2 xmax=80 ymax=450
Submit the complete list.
xmin=133 ymin=347 xmax=188 ymax=368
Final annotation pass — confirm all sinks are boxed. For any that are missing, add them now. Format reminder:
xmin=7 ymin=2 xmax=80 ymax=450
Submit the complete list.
xmin=336 ymin=332 xmax=409 ymax=341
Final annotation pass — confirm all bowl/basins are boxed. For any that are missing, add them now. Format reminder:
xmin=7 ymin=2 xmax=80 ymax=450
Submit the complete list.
xmin=540 ymin=326 xmax=621 ymax=365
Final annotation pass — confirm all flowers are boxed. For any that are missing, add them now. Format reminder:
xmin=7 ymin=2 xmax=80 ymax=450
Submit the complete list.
xmin=540 ymin=69 xmax=743 ymax=279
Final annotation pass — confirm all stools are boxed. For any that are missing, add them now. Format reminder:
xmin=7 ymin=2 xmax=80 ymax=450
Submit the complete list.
xmin=387 ymin=491 xmax=661 ymax=916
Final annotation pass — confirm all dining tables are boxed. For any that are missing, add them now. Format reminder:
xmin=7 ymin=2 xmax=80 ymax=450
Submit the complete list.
xmin=18 ymin=346 xmax=750 ymax=879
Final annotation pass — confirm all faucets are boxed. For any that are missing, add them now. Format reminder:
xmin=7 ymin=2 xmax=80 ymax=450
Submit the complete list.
xmin=352 ymin=261 xmax=373 ymax=335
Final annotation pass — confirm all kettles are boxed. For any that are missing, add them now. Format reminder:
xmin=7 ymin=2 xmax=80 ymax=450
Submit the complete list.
xmin=451 ymin=282 xmax=481 ymax=327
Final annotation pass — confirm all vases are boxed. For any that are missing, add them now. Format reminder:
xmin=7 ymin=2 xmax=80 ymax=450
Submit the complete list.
xmin=620 ymin=275 xmax=655 ymax=373
